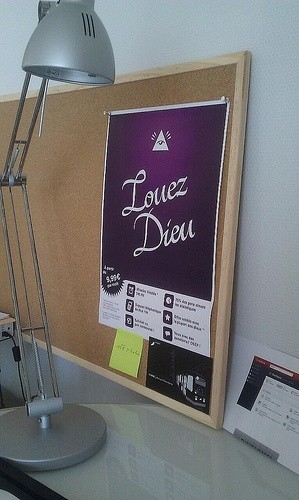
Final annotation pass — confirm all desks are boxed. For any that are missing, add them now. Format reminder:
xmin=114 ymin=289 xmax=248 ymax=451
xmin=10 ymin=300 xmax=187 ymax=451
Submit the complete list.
xmin=0 ymin=402 xmax=299 ymax=500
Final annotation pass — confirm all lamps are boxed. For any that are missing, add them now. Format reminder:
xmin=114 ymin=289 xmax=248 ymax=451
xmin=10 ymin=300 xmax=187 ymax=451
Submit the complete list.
xmin=0 ymin=0 xmax=118 ymax=474
